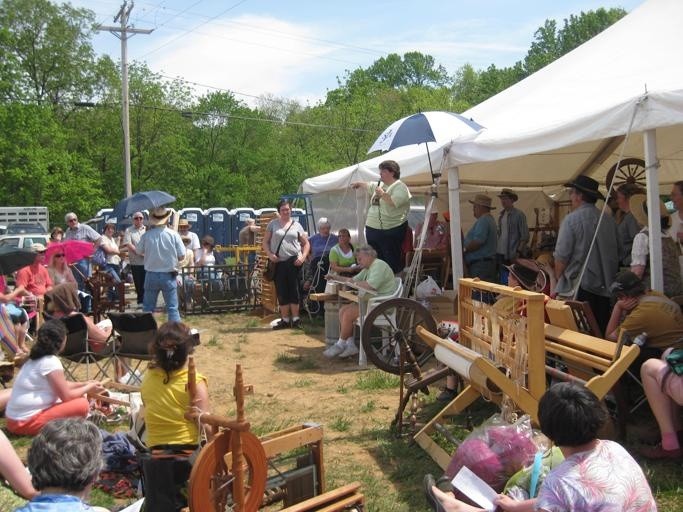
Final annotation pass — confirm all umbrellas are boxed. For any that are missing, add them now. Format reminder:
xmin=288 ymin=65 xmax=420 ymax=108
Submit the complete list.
xmin=368 ymin=105 xmax=485 ymax=195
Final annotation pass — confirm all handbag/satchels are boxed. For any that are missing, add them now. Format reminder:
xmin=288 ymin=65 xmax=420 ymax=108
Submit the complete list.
xmin=262 ymin=260 xmax=277 ymax=282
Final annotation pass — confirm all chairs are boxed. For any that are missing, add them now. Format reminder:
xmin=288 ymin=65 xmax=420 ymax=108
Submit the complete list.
xmin=352 ymin=278 xmax=405 ymax=366
xmin=0 ymin=264 xmax=201 ymax=392
xmin=558 ymin=300 xmax=648 ymax=443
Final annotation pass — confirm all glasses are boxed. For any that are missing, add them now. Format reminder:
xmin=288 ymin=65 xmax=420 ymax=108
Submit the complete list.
xmin=54 ymin=253 xmax=65 ymax=258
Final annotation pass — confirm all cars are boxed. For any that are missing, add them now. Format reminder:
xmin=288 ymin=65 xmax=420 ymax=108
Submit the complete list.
xmin=0 ymin=233 xmax=61 ymax=250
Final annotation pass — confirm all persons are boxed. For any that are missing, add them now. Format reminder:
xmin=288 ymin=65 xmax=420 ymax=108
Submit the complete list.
xmin=176 ymin=220 xmax=223 ymax=310
xmin=264 ymin=200 xmax=310 ymax=329
xmin=1 ymin=243 xmax=141 ymax=411
xmin=6 ymin=319 xmax=105 ymax=435
xmin=99 ymin=212 xmax=149 ymax=300
xmin=606 ymin=271 xmax=683 ymax=406
xmin=16 ymin=417 xmax=107 ymax=511
xmin=239 ymin=220 xmax=261 ymax=274
xmin=329 ymin=229 xmax=361 ymax=279
xmin=301 ymin=221 xmax=338 ymax=314
xmin=52 ymin=213 xmax=102 ymax=315
xmin=415 ymin=191 xmax=530 ymax=307
xmin=136 ymin=208 xmax=187 ymax=322
xmin=365 ymin=161 xmax=411 ymax=273
xmin=617 ymin=182 xmax=682 ymax=299
xmin=436 ymin=253 xmax=555 ymax=399
xmin=423 ymin=381 xmax=656 ymax=512
xmin=553 ymin=176 xmax=617 ymax=335
xmin=141 ymin=322 xmax=228 ymax=512
xmin=639 ymin=348 xmax=682 ymax=458
xmin=324 ymin=247 xmax=397 ymax=358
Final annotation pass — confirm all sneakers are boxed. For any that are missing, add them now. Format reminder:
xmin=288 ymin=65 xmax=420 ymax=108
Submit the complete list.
xmin=339 ymin=345 xmax=360 ymax=360
xmin=320 ymin=344 xmax=345 ymax=360
xmin=273 ymin=320 xmax=291 ymax=330
xmin=292 ymin=320 xmax=304 ymax=329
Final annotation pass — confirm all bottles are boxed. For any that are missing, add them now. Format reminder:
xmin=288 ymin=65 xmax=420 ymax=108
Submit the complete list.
xmin=632 ymin=331 xmax=648 ymax=348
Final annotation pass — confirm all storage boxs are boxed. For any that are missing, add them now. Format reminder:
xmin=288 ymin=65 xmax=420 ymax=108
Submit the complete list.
xmin=425 ymin=290 xmax=458 ymax=323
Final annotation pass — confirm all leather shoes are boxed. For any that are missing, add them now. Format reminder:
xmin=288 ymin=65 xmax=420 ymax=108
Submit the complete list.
xmin=422 ymin=472 xmax=456 ymax=511
xmin=638 ymin=442 xmax=682 ymax=459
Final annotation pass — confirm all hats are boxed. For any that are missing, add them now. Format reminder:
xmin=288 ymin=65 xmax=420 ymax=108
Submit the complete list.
xmin=468 ymin=194 xmax=497 ymax=210
xmin=562 ymin=174 xmax=605 ymax=201
xmin=177 ymin=234 xmax=192 ymax=245
xmin=178 ymin=218 xmax=193 ymax=230
xmin=497 ymin=187 xmax=518 ymax=201
xmin=610 ymin=268 xmax=642 ymax=292
xmin=31 ymin=243 xmax=47 ymax=253
xmin=429 ymin=206 xmax=450 ymax=222
xmin=501 ymin=256 xmax=544 ymax=291
xmin=628 ymin=191 xmax=674 ymax=232
xmin=147 ymin=204 xmax=172 ymax=226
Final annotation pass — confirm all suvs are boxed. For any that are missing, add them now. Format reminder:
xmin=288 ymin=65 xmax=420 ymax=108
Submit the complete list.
xmin=6 ymin=223 xmax=46 ymax=233
